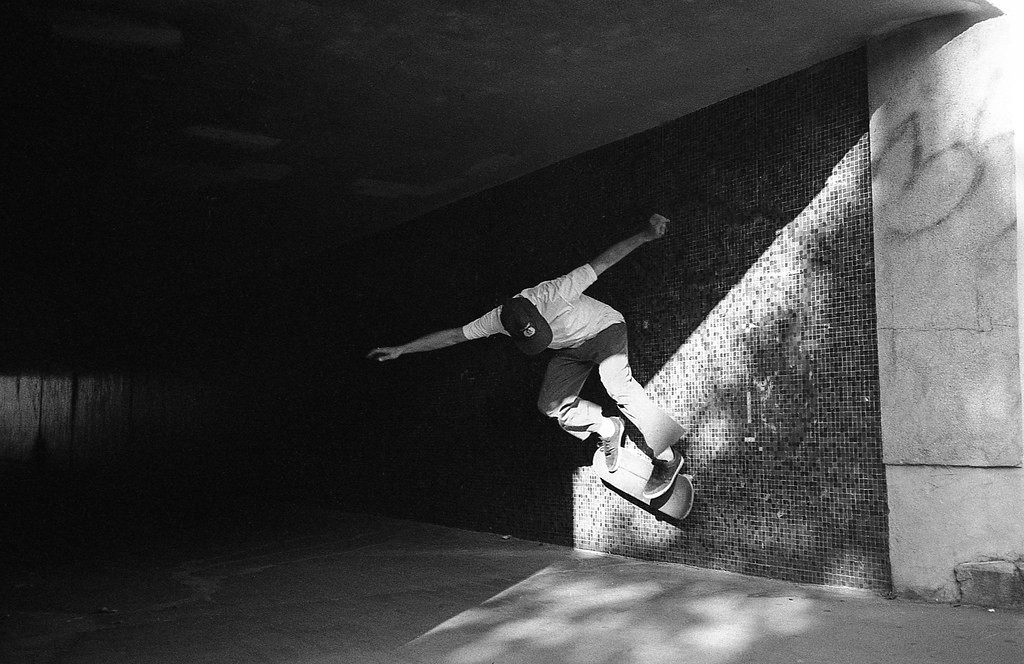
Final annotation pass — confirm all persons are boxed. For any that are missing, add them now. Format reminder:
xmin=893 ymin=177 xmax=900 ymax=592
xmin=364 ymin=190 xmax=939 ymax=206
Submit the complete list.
xmin=365 ymin=215 xmax=685 ymax=498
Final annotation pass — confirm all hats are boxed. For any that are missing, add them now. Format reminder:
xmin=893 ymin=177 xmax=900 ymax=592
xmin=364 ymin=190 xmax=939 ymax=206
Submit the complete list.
xmin=500 ymin=297 xmax=553 ymax=354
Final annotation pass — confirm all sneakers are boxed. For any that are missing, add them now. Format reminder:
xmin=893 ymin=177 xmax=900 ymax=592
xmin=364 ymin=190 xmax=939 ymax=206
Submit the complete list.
xmin=596 ymin=415 xmax=624 ymax=472
xmin=643 ymin=447 xmax=684 ymax=499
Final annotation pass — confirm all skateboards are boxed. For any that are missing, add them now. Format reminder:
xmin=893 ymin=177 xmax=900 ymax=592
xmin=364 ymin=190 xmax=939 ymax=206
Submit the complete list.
xmin=593 ymin=445 xmax=694 ymax=520
